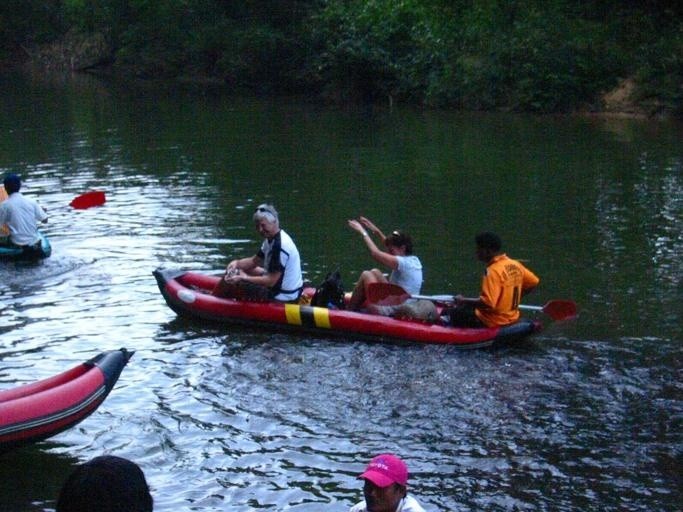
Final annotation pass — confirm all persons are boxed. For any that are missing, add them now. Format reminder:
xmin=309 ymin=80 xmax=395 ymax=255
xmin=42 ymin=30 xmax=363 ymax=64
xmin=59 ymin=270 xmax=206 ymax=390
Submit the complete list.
xmin=342 ymin=215 xmax=424 ymax=312
xmin=449 ymin=230 xmax=540 ymax=328
xmin=212 ymin=204 xmax=305 ymax=304
xmin=0 ymin=174 xmax=50 ymax=254
xmin=55 ymin=456 xmax=154 ymax=512
xmin=348 ymin=453 xmax=426 ymax=512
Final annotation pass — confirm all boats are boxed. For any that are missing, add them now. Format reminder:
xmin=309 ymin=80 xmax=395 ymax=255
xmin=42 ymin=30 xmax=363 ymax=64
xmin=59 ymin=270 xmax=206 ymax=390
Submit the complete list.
xmin=0 ymin=232 xmax=52 ymax=262
xmin=0 ymin=346 xmax=137 ymax=453
xmin=152 ymin=265 xmax=543 ymax=349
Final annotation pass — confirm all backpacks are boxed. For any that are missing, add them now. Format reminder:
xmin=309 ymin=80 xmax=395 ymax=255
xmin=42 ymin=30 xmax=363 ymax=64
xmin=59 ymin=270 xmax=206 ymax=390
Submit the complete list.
xmin=310 ymin=268 xmax=346 ymax=307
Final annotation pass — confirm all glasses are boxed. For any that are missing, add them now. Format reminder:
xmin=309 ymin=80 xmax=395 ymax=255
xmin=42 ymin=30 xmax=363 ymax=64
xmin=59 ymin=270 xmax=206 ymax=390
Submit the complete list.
xmin=254 ymin=208 xmax=274 ymax=216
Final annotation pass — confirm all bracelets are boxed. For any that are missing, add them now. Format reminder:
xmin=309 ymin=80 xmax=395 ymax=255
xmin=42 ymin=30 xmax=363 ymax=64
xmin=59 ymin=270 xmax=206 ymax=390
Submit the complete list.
xmin=361 ymin=232 xmax=368 ymax=236
